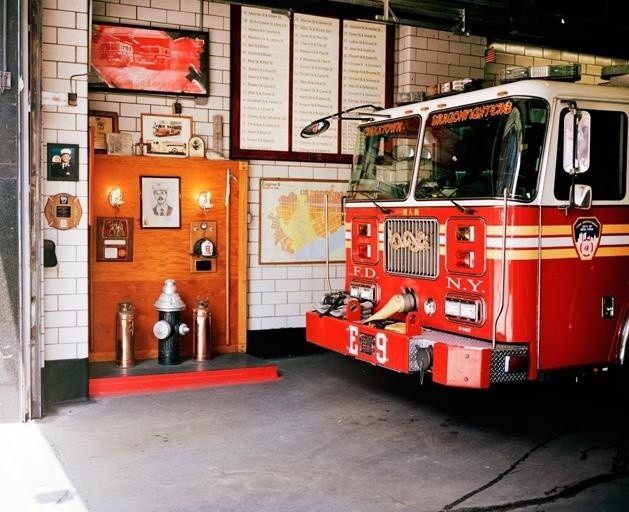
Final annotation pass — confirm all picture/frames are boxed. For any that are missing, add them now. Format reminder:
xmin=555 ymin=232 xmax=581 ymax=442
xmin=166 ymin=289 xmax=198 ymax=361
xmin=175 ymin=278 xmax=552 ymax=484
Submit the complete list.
xmin=138 ymin=175 xmax=182 ymax=230
xmin=257 ymin=177 xmax=351 ymax=265
xmin=47 ymin=142 xmax=80 ymax=181
xmin=140 ymin=112 xmax=194 ymax=158
xmin=88 ymin=109 xmax=120 ymax=154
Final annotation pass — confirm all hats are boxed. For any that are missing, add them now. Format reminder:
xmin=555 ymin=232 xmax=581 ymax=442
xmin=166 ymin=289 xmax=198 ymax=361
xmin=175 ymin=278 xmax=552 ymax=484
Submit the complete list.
xmin=60 ymin=149 xmax=72 ymax=156
xmin=189 ymin=238 xmax=219 ymax=258
xmin=153 ymin=183 xmax=168 ymax=193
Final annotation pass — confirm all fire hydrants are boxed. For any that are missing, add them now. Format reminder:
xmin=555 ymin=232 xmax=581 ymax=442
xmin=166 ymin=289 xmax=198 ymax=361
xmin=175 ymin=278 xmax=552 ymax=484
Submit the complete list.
xmin=151 ymin=278 xmax=190 ymax=365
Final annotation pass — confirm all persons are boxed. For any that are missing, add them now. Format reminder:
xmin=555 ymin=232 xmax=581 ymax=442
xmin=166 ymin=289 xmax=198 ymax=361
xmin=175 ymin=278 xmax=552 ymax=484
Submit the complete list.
xmin=51 ymin=149 xmax=76 ymax=178
xmin=152 ymin=182 xmax=173 ymax=216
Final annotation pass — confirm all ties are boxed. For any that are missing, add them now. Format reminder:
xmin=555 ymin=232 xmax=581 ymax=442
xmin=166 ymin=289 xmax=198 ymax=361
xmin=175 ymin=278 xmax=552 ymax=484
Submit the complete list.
xmin=160 ymin=209 xmax=164 ymax=216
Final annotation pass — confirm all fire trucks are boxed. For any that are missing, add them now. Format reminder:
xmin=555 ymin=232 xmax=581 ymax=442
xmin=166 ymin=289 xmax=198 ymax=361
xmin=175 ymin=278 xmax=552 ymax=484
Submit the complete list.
xmin=300 ymin=62 xmax=629 ymax=388
